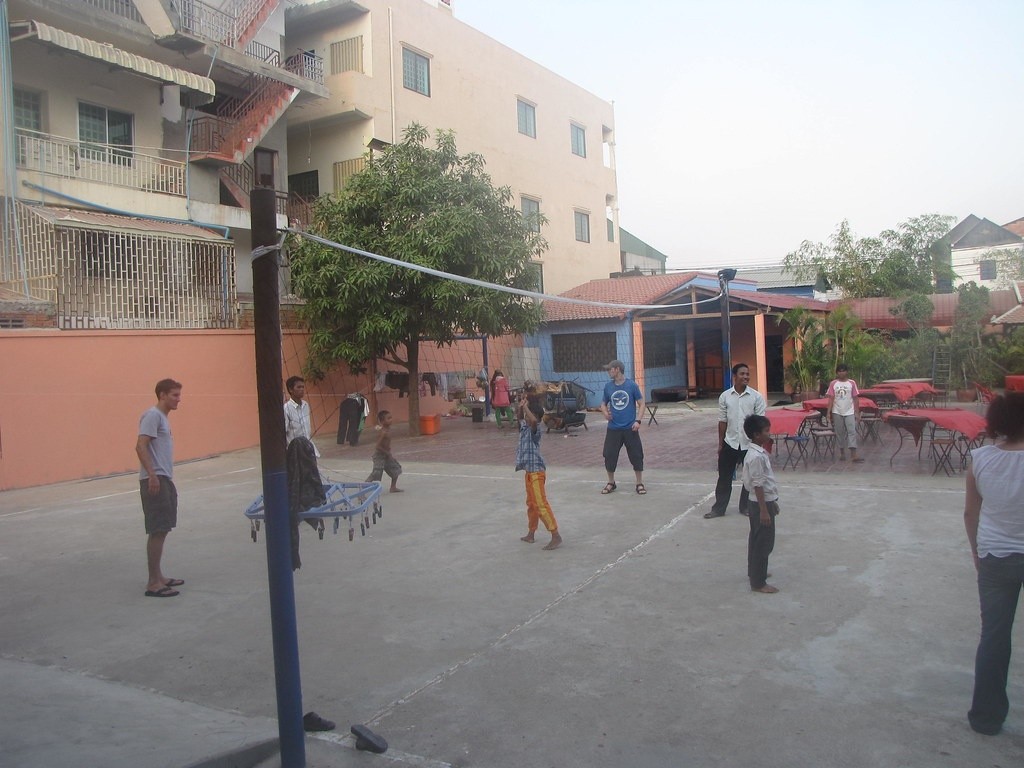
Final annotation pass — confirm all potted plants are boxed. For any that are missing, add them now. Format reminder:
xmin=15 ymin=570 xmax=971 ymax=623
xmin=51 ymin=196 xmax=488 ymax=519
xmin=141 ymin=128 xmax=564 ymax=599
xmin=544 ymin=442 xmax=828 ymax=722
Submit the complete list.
xmin=780 ymin=307 xmax=830 ymax=404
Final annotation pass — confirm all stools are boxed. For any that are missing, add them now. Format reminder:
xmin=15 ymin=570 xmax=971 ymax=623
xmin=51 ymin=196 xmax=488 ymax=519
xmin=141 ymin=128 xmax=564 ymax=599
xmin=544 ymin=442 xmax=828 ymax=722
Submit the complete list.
xmin=763 ymin=380 xmax=996 ymax=477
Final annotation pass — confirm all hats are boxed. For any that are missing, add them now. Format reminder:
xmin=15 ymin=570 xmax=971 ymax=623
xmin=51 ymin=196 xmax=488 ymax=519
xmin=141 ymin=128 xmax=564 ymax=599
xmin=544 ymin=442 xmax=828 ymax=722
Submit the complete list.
xmin=602 ymin=360 xmax=624 ymax=371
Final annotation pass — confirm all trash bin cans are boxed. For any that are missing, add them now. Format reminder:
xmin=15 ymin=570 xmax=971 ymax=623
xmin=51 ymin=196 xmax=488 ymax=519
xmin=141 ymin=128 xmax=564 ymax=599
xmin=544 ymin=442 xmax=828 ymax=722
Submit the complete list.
xmin=420 ymin=413 xmax=442 ymax=435
xmin=472 ymin=408 xmax=483 ymax=422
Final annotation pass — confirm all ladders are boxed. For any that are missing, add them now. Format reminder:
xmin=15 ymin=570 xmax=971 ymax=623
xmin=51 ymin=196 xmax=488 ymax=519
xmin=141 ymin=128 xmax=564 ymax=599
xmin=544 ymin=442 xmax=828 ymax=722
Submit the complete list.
xmin=931 ymin=344 xmax=953 ymax=408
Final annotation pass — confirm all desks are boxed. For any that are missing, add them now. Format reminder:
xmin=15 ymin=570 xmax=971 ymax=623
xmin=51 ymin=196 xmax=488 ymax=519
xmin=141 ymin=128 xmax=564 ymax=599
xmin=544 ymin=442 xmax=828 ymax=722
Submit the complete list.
xmin=872 ymin=381 xmax=936 ymax=407
xmin=883 ymin=408 xmax=987 ymax=476
xmin=803 ymin=397 xmax=877 ymax=441
xmin=764 ymin=406 xmax=823 ymax=471
xmin=856 ymin=389 xmax=911 ymax=408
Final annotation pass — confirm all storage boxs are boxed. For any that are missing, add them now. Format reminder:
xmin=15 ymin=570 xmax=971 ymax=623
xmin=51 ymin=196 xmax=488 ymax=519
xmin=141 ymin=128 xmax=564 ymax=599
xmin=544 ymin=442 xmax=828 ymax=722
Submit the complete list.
xmin=420 ymin=413 xmax=441 ymax=435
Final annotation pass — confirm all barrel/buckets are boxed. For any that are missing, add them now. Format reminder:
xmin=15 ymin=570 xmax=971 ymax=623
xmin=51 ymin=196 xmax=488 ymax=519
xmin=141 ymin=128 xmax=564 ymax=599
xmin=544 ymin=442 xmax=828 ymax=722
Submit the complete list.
xmin=472 ymin=407 xmax=483 ymax=422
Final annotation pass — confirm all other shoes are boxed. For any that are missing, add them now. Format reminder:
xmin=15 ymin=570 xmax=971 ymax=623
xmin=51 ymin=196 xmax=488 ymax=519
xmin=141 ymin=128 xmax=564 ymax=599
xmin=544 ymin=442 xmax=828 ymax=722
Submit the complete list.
xmin=740 ymin=510 xmax=749 ymax=516
xmin=705 ymin=511 xmax=724 ymax=518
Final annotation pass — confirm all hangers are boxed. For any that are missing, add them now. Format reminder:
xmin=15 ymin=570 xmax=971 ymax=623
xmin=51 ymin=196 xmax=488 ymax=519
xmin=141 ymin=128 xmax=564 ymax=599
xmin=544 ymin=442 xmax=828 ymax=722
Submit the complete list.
xmin=348 ymin=391 xmax=364 ymax=401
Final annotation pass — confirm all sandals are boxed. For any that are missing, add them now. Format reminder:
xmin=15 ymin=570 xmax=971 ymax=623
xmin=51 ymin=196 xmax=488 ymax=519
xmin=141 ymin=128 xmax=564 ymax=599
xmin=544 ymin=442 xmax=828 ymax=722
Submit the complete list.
xmin=601 ymin=481 xmax=616 ymax=494
xmin=636 ymin=484 xmax=647 ymax=494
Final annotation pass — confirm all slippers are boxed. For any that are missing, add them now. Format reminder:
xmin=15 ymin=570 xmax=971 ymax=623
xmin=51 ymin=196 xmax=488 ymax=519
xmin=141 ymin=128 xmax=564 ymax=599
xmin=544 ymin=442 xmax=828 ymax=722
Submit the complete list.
xmin=145 ymin=587 xmax=179 ymax=597
xmin=164 ymin=579 xmax=184 ymax=586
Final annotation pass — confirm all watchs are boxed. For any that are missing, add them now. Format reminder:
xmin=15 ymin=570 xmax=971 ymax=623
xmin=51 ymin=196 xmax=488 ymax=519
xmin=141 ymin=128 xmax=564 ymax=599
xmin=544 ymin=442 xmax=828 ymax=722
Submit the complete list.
xmin=634 ymin=420 xmax=641 ymax=424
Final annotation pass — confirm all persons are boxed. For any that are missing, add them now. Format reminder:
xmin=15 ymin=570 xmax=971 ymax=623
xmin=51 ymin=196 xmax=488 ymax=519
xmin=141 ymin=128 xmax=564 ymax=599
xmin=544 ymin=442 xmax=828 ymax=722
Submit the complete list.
xmin=516 ymin=399 xmax=562 ymax=550
xmin=703 ymin=363 xmax=767 ymax=520
xmin=601 ymin=359 xmax=647 ymax=494
xmin=825 ymin=364 xmax=865 ymax=461
xmin=490 ymin=370 xmax=518 ymax=429
xmin=284 ymin=377 xmax=321 ymax=459
xmin=742 ymin=415 xmax=780 ymax=594
xmin=964 ymin=390 xmax=1024 ymax=735
xmin=365 ymin=410 xmax=404 ymax=492
xmin=134 ymin=379 xmax=185 ymax=596
